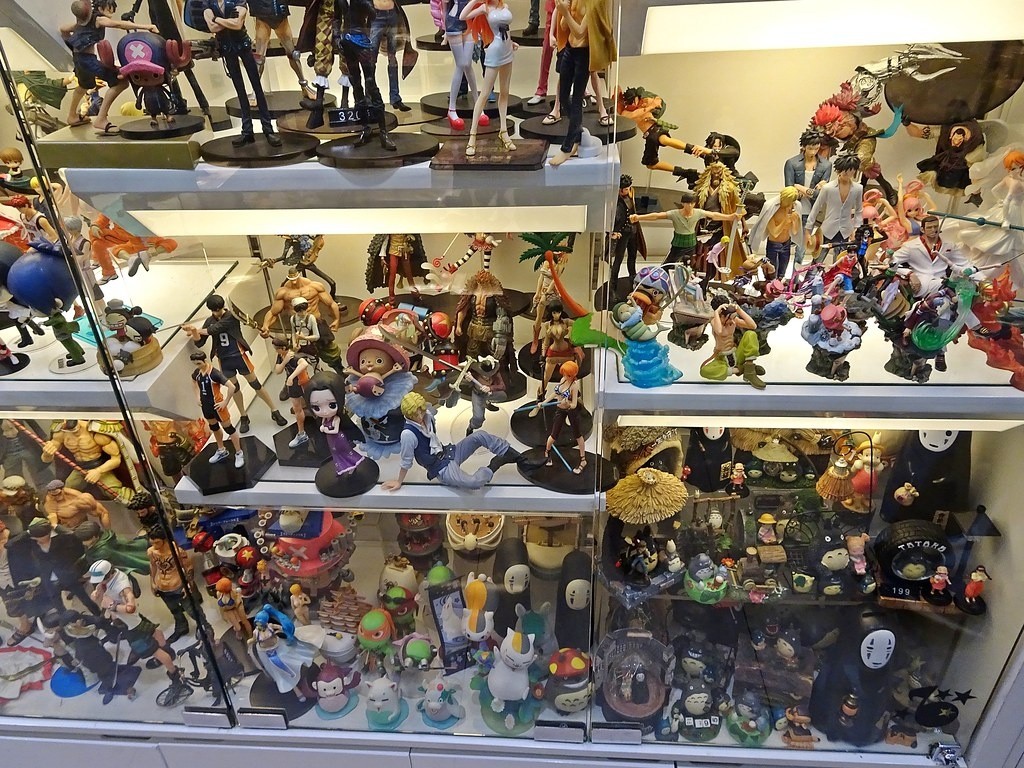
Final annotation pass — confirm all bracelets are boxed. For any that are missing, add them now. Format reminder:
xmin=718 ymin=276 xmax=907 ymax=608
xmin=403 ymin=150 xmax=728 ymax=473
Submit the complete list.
xmin=567 ymin=404 xmax=571 ymax=410
xmin=107 ymin=600 xmax=120 ymax=613
xmin=212 ymin=16 xmax=217 ymax=22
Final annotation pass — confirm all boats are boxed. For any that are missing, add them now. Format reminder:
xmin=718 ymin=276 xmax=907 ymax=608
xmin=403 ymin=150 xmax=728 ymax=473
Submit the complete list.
xmin=670 ymin=301 xmax=715 ymax=323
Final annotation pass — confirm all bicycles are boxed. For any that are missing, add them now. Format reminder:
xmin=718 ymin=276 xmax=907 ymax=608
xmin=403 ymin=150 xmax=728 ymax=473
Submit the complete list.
xmin=156 ymin=651 xmax=246 ymax=708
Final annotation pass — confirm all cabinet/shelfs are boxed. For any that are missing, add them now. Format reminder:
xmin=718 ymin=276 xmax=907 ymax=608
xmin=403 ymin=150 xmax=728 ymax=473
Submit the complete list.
xmin=2 ymin=2 xmax=1023 ymax=768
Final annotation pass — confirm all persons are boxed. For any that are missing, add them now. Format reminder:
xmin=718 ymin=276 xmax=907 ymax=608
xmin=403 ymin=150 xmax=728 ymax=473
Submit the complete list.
xmin=609 ymin=87 xmax=1024 ymax=702
xmin=0 ymin=0 xmax=618 ymax=701
